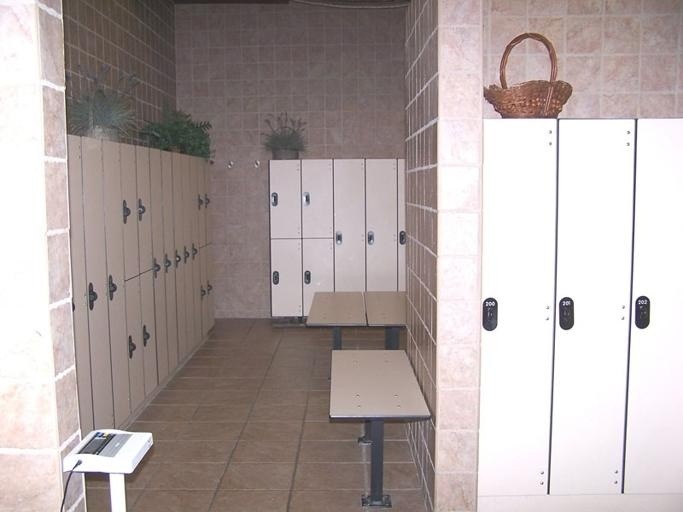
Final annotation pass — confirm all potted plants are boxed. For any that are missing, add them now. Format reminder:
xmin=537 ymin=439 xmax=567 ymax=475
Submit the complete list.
xmin=71 ymin=64 xmax=151 ymax=141
xmin=142 ymin=102 xmax=213 ymax=153
xmin=260 ymin=111 xmax=307 ymax=160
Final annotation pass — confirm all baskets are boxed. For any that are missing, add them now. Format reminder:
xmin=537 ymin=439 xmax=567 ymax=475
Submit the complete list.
xmin=482 ymin=32 xmax=573 ymax=118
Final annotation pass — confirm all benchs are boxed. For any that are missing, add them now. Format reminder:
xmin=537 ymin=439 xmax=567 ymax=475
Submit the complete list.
xmin=329 ymin=350 xmax=431 ymax=508
xmin=306 ymin=291 xmax=367 ymax=351
xmin=363 ymin=290 xmax=406 ymax=351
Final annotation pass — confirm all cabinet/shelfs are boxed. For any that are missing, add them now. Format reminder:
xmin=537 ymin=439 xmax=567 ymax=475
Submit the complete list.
xmin=268 ymin=159 xmax=405 ymax=329
xmin=64 ymin=135 xmax=217 ymax=439
xmin=479 ymin=117 xmax=682 ymax=496
